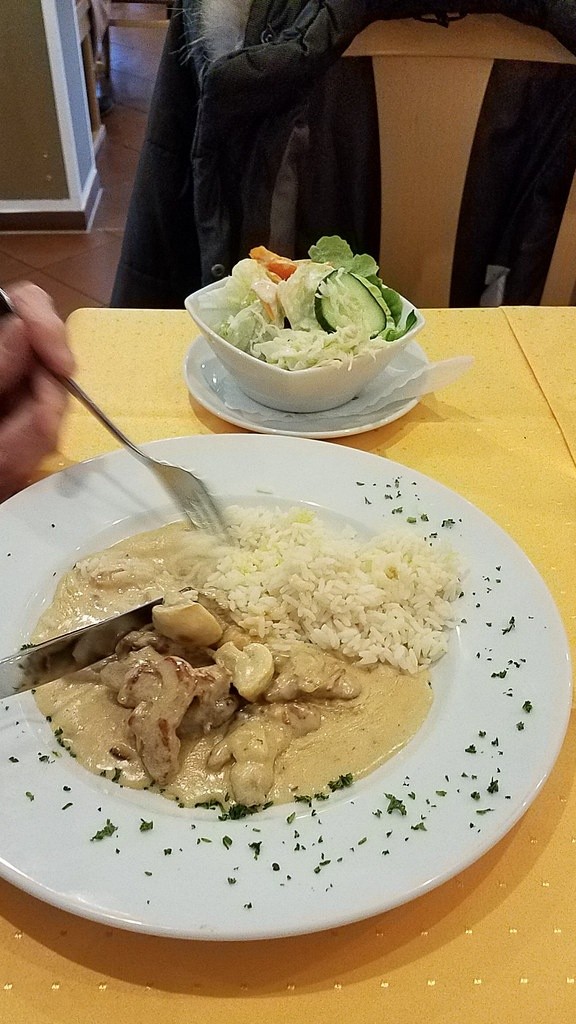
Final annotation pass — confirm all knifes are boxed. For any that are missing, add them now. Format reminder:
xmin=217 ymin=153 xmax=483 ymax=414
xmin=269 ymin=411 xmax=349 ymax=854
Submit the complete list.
xmin=0 ymin=584 xmax=194 ymax=707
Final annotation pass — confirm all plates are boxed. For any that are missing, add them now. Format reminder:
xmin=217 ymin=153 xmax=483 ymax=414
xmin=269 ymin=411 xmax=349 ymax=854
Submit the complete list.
xmin=185 ymin=332 xmax=430 ymax=438
xmin=0 ymin=434 xmax=572 ymax=946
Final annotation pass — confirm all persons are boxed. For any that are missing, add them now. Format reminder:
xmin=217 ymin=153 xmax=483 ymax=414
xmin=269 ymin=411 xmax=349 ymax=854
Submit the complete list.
xmin=0 ymin=278 xmax=76 ymax=501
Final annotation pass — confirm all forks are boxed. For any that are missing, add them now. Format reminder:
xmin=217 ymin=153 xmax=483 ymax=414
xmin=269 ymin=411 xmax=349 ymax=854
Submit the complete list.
xmin=2 ymin=286 xmax=238 ymax=551
xmin=0 ymin=285 xmax=237 ymax=547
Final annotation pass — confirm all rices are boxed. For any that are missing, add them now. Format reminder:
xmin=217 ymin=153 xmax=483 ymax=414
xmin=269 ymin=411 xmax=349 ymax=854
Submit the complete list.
xmin=205 ymin=504 xmax=461 ymax=673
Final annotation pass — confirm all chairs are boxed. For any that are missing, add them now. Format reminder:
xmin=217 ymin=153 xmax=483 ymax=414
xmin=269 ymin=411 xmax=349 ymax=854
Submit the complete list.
xmin=340 ymin=13 xmax=576 ymax=306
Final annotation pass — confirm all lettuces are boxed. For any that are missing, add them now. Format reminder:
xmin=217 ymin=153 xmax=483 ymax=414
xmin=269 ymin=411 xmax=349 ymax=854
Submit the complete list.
xmin=196 ymin=235 xmax=418 ymax=370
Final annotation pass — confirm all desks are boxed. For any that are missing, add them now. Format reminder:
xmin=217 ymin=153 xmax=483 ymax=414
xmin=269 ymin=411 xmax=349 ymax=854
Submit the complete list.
xmin=1 ymin=307 xmax=574 ymax=1021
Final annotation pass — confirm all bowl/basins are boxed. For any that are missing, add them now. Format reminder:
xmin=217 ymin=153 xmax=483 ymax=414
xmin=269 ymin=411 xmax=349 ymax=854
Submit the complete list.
xmin=185 ymin=267 xmax=426 ymax=414
